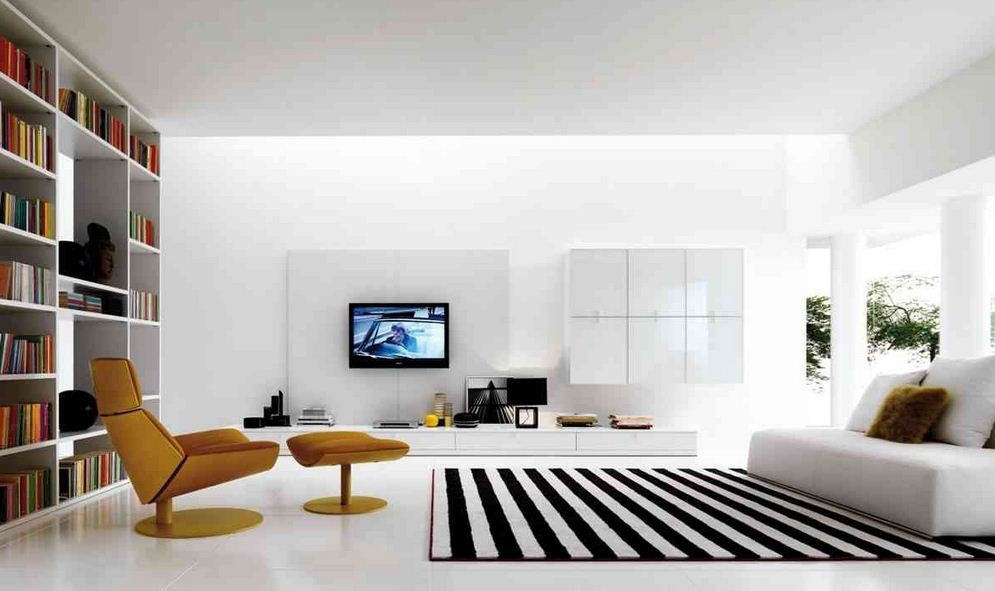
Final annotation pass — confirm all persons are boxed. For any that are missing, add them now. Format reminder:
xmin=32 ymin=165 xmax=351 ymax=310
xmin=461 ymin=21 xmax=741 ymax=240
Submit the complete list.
xmin=386 ymin=325 xmax=416 ymax=352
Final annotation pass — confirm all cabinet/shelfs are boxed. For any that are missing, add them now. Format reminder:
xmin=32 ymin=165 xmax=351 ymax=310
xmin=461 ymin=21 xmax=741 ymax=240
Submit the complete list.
xmin=0 ymin=0 xmax=166 ymax=540
xmin=224 ymin=422 xmax=699 ymax=458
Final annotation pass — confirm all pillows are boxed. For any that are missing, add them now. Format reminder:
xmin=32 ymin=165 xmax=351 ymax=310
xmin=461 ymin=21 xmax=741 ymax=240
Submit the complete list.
xmin=864 ymin=382 xmax=949 ymax=444
xmin=845 ymin=372 xmax=929 ymax=433
xmin=920 ymin=353 xmax=995 ymax=448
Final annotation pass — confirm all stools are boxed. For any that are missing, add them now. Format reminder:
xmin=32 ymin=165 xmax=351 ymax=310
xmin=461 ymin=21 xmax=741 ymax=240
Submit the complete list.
xmin=286 ymin=431 xmax=410 ymax=515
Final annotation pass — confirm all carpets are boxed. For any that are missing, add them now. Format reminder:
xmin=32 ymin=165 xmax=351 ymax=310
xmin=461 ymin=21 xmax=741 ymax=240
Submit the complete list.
xmin=429 ymin=466 xmax=995 ymax=562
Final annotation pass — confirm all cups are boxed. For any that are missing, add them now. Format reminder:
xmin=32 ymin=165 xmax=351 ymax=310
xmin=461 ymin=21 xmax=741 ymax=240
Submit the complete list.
xmin=424 ymin=411 xmax=439 ymax=427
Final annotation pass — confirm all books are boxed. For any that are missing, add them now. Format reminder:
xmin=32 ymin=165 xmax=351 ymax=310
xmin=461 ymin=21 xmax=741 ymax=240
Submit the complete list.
xmin=0 ymin=38 xmax=55 ymax=528
xmin=58 ymin=287 xmax=158 ymax=322
xmin=59 ymin=447 xmax=126 ymax=500
xmin=130 ymin=210 xmax=155 ymax=247
xmin=59 ymin=88 xmax=160 ymax=177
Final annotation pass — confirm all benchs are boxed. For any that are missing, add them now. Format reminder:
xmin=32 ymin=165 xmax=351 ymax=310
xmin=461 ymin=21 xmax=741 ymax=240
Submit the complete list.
xmin=746 ymin=420 xmax=995 ymax=537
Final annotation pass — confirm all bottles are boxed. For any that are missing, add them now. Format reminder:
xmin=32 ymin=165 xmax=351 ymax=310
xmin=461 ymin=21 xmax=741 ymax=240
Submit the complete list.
xmin=434 ymin=392 xmax=453 ymax=427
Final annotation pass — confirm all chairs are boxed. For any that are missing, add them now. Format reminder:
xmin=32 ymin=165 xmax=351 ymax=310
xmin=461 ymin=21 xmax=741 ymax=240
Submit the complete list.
xmin=88 ymin=357 xmax=279 ymax=540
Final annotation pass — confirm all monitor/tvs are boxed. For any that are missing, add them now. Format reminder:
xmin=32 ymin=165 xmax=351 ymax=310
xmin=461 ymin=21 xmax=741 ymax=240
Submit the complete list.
xmin=348 ymin=302 xmax=450 ymax=369
